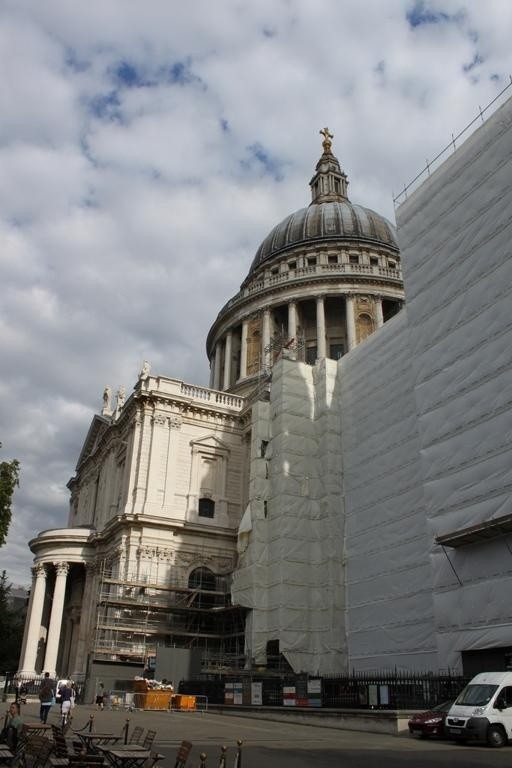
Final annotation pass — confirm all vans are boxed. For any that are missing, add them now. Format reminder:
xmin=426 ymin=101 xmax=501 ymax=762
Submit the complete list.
xmin=55 ymin=679 xmax=77 ymax=703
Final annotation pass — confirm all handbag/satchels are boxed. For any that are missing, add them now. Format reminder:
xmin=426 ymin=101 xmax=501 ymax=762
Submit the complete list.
xmin=51 ymin=697 xmax=56 ymax=706
xmin=69 ymin=696 xmax=75 ymax=709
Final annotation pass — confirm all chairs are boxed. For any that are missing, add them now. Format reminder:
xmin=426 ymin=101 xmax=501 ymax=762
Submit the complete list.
xmin=0 ymin=711 xmax=192 ymax=768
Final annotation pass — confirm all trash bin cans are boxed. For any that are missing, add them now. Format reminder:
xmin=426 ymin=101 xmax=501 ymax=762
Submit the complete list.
xmin=176 ymin=695 xmax=196 ymax=712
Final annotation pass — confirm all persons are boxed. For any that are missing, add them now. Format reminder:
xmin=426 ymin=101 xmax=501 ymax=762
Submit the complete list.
xmin=5 ymin=701 xmax=25 ymax=737
xmin=92 ymin=682 xmax=105 ymax=712
xmin=36 ymin=672 xmax=79 ymax=726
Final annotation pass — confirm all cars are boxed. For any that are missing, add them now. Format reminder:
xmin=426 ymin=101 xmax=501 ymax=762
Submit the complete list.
xmin=408 ymin=699 xmax=458 ymax=739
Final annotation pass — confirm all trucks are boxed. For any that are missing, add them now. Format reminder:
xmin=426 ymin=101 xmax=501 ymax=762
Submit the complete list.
xmin=440 ymin=670 xmax=511 ymax=748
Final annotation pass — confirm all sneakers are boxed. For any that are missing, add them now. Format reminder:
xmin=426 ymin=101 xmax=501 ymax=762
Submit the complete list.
xmin=40 ymin=719 xmax=47 ymax=724
